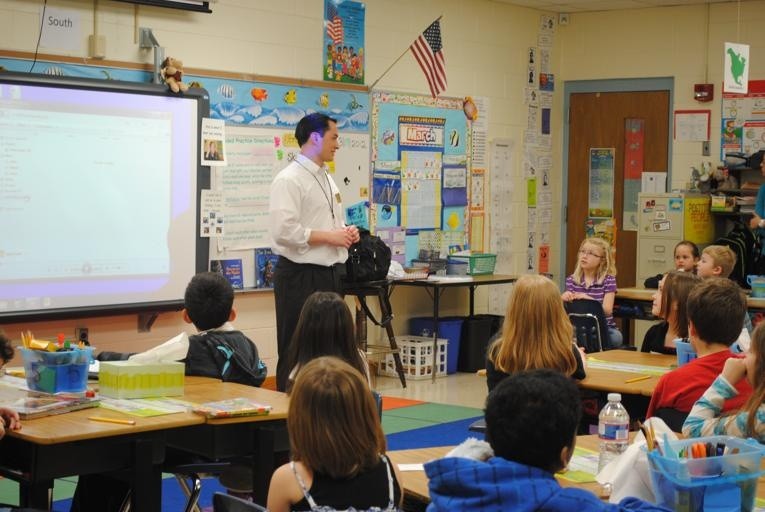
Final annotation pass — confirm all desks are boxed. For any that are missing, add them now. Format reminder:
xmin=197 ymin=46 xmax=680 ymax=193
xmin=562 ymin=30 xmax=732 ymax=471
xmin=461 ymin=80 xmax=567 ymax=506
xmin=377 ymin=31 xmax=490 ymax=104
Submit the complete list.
xmin=386 ymin=431 xmax=638 ymax=505
xmin=86 ymin=376 xmax=223 ymax=392
xmin=397 ymin=275 xmax=518 ymax=383
xmin=6 ymin=406 xmax=205 ymax=512
xmin=640 ymin=380 xmax=661 ymax=396
xmin=615 ymin=288 xmax=765 ymax=349
xmin=169 ymin=383 xmax=291 ymax=508
xmin=578 ymin=351 xmax=679 ymax=395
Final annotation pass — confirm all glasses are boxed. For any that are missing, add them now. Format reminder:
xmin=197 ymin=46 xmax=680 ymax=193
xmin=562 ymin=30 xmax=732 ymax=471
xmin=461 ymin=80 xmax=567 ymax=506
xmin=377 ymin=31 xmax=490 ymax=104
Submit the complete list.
xmin=579 ymin=249 xmax=605 ymax=259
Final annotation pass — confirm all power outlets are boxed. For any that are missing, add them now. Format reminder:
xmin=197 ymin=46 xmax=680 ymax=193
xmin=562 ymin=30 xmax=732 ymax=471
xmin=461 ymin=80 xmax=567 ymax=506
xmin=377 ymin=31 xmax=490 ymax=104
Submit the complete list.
xmin=703 ymin=141 xmax=710 ymax=155
xmin=74 ymin=328 xmax=88 ymax=344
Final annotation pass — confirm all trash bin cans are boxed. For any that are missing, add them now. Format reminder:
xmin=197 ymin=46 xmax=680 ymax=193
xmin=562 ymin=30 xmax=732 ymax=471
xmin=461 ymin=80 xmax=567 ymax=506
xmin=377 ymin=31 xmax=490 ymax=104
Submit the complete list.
xmin=412 ymin=316 xmax=462 ymax=372
xmin=459 ymin=315 xmax=507 ymax=372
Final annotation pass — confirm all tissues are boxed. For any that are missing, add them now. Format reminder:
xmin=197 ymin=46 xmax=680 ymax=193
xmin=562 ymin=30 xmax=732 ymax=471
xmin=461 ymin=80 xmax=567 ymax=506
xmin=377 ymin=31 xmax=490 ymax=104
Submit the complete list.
xmin=99 ymin=331 xmax=190 ymax=400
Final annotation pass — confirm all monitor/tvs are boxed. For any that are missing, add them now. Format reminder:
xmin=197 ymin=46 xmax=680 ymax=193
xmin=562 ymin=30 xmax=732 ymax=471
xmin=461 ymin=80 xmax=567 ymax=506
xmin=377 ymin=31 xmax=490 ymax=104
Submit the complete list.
xmin=0 ymin=70 xmax=210 ymax=324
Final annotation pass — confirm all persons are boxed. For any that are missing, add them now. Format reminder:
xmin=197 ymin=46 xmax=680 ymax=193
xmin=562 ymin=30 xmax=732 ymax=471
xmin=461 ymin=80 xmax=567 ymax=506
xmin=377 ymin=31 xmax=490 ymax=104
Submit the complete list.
xmin=130 ymin=272 xmax=265 ymax=512
xmin=671 ymin=242 xmax=693 ymax=273
xmin=487 ymin=272 xmax=591 ymax=436
xmin=424 ymin=371 xmax=670 ymax=512
xmin=697 ymin=243 xmax=753 ymax=333
xmin=0 ymin=334 xmax=21 ymax=442
xmin=678 ymin=321 xmax=765 ymax=444
xmin=254 ymin=289 xmax=372 ymax=507
xmin=269 ymin=113 xmax=360 ymax=392
xmin=556 ymin=236 xmax=621 ymax=349
xmin=205 ymin=142 xmax=220 ymax=161
xmin=382 ymin=206 xmax=392 ymax=219
xmin=749 ymin=153 xmax=765 ymax=277
xmin=326 ymin=45 xmax=363 ymax=80
xmin=265 ymin=357 xmax=404 ymax=512
xmin=641 ymin=271 xmax=699 ymax=357
xmin=644 ymin=284 xmax=756 ymax=432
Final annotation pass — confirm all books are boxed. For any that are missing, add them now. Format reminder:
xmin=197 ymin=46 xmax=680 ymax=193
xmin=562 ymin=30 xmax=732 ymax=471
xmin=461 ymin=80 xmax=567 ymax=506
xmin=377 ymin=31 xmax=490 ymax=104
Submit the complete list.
xmin=255 ymin=248 xmax=279 ymax=289
xmin=211 ymin=259 xmax=243 ymax=291
xmin=735 ymin=195 xmax=757 ymax=213
xmin=711 ymin=206 xmax=733 ymax=212
xmin=88 ymin=358 xmax=100 ymax=380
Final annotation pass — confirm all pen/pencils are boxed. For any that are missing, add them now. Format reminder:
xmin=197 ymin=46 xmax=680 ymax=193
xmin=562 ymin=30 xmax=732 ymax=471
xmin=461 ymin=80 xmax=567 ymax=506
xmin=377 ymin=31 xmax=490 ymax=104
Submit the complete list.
xmin=624 ymin=374 xmax=655 ymax=383
xmin=640 ymin=420 xmax=737 ymax=477
xmin=89 ymin=416 xmax=136 ymax=428
xmin=20 ymin=329 xmax=87 ymax=366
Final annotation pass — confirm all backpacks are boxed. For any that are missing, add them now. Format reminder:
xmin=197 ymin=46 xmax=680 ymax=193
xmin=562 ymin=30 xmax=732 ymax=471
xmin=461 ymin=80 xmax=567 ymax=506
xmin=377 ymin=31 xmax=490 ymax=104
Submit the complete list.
xmin=715 ymin=222 xmax=754 ymax=285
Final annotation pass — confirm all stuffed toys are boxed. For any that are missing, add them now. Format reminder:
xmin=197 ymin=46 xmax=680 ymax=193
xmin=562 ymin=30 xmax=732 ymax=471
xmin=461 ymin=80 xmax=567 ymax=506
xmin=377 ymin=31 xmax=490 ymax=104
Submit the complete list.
xmin=161 ymin=57 xmax=189 ymax=93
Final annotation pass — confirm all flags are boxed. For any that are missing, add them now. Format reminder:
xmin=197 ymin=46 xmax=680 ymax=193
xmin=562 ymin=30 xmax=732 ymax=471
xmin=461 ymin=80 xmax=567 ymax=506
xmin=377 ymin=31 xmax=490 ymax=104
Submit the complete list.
xmin=407 ymin=15 xmax=450 ymax=101
xmin=325 ymin=1 xmax=344 ymax=45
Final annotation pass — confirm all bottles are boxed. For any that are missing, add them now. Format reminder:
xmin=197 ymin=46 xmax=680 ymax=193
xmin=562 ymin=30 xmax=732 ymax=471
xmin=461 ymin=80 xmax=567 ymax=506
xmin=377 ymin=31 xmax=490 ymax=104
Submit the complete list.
xmin=597 ymin=393 xmax=630 ymax=498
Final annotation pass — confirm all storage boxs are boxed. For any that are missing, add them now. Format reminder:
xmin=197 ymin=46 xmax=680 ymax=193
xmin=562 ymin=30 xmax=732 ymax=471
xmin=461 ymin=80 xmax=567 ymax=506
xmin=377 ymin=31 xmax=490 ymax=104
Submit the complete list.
xmin=375 ymin=336 xmax=449 ymax=381
